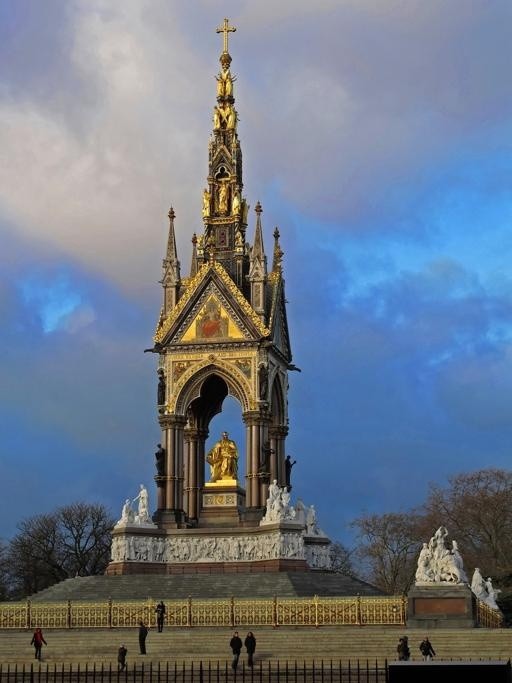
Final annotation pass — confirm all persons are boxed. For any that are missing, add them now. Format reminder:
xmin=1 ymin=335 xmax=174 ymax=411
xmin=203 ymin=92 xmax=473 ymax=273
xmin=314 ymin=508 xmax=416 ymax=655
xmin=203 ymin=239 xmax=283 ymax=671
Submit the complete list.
xmin=133 ymin=483 xmax=149 ymax=515
xmin=122 ymin=499 xmax=136 ymax=522
xmin=157 ymin=376 xmax=166 ymax=404
xmin=200 ymin=306 xmax=221 ymax=320
xmin=138 ymin=621 xmax=148 ymax=654
xmin=30 ymin=627 xmax=47 ymax=658
xmin=265 ymin=479 xmax=318 ymax=530
xmin=285 ymin=455 xmax=297 ymax=486
xmin=154 ymin=443 xmax=165 ymax=474
xmin=230 ymin=631 xmax=242 ymax=669
xmin=397 ymin=636 xmax=410 ymax=657
xmin=117 ymin=643 xmax=128 ymax=672
xmin=110 ymin=531 xmax=332 ymax=570
xmin=154 ymin=600 xmax=165 ymax=632
xmin=244 ymin=631 xmax=256 ymax=666
xmin=415 ymin=525 xmax=501 ymax=612
xmin=397 ymin=638 xmax=409 ymax=661
xmin=215 ymin=430 xmax=238 ymax=476
xmin=419 ymin=636 xmax=436 ymax=661
xmin=197 ymin=68 xmax=251 ymax=255
